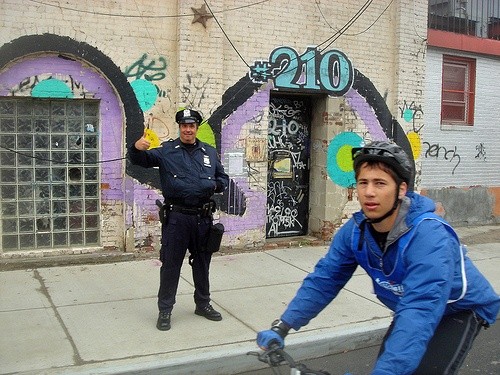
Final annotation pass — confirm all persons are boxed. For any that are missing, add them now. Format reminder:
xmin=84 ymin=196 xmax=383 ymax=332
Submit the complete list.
xmin=128 ymin=108 xmax=229 ymax=331
xmin=257 ymin=140 xmax=500 ymax=375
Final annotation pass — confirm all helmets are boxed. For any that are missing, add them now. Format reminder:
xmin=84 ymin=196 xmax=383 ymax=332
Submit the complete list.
xmin=352 ymin=140 xmax=412 ymax=187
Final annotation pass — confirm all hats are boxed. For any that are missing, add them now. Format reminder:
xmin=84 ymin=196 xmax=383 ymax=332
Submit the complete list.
xmin=175 ymin=109 xmax=202 ymax=124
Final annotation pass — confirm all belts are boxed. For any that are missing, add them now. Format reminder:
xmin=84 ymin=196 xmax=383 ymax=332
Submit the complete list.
xmin=169 ymin=204 xmax=203 ymax=215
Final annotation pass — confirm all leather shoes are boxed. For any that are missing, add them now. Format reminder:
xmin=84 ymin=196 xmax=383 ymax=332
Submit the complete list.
xmin=195 ymin=302 xmax=222 ymax=321
xmin=156 ymin=311 xmax=171 ymax=331
